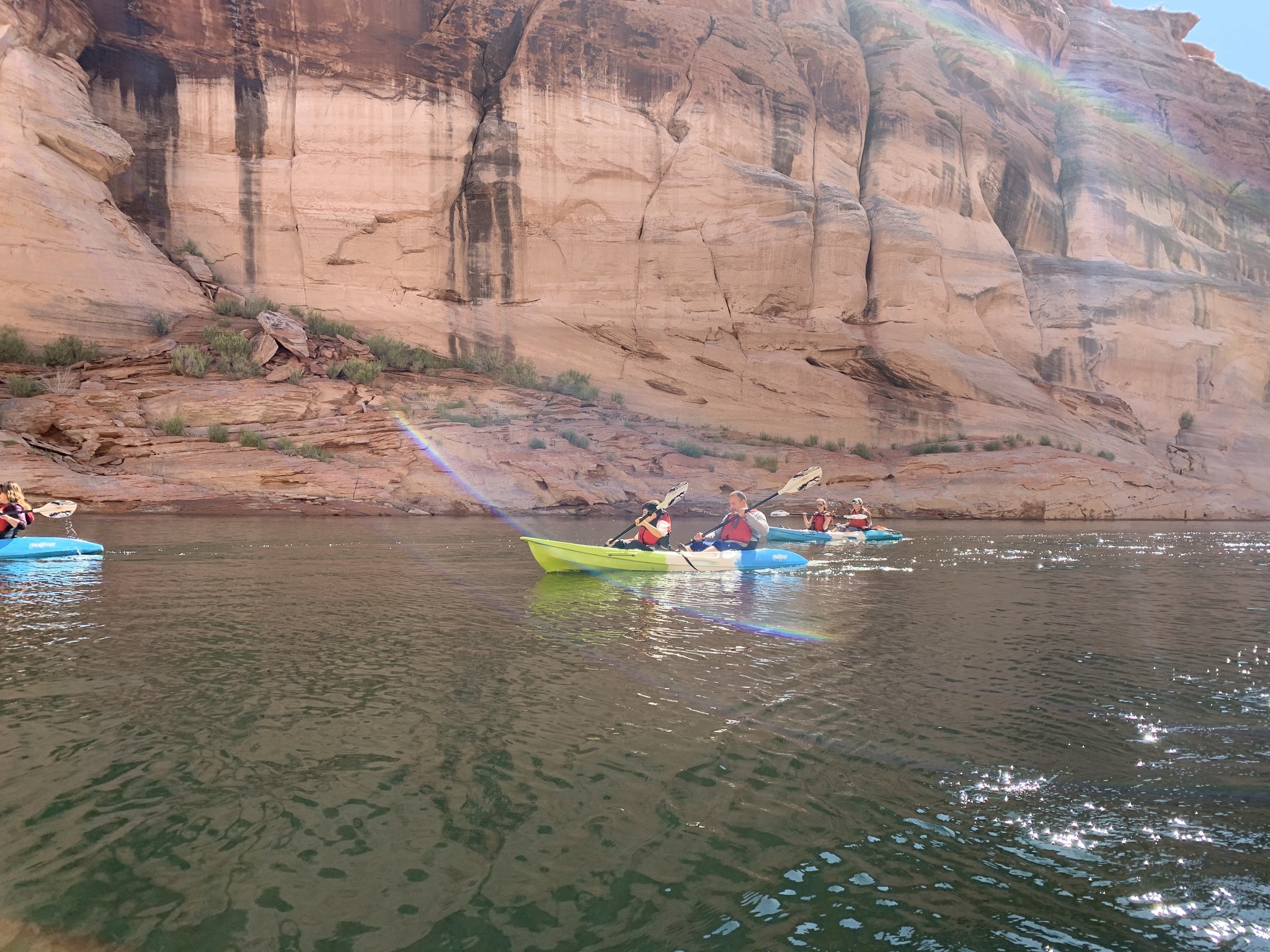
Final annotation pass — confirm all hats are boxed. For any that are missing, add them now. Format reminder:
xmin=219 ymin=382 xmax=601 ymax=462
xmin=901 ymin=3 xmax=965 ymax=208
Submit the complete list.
xmin=852 ymin=497 xmax=863 ymax=504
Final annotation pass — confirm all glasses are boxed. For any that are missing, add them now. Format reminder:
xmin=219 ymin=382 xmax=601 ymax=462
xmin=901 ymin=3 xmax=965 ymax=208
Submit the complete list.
xmin=816 ymin=503 xmax=824 ymax=506
xmin=852 ymin=501 xmax=860 ymax=505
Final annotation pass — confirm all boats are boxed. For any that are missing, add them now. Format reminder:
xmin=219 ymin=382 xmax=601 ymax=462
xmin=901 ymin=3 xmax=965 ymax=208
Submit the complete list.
xmin=766 ymin=523 xmax=904 ymax=542
xmin=0 ymin=536 xmax=104 ymax=558
xmin=518 ymin=533 xmax=810 ymax=573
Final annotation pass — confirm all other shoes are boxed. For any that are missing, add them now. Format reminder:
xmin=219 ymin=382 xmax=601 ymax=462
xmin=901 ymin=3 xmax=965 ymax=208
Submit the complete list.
xmin=786 ymin=526 xmax=791 ymax=530
xmin=779 ymin=525 xmax=784 ymax=528
xmin=678 ymin=543 xmax=687 ymax=552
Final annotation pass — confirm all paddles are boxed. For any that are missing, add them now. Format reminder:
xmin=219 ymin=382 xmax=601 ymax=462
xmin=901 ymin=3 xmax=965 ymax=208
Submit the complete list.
xmin=0 ymin=500 xmax=78 ymax=518
xmin=685 ymin=463 xmax=828 ymax=551
xmin=769 ymin=509 xmax=869 ymax=521
xmin=608 ymin=480 xmax=688 ymax=549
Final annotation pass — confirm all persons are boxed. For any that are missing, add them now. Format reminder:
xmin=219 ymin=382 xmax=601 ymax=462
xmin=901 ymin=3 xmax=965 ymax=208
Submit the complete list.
xmin=0 ymin=481 xmax=34 ymax=539
xmin=828 ymin=498 xmax=872 ymax=532
xmin=779 ymin=498 xmax=833 ymax=532
xmin=606 ymin=500 xmax=671 ymax=552
xmin=678 ymin=491 xmax=770 ymax=552
